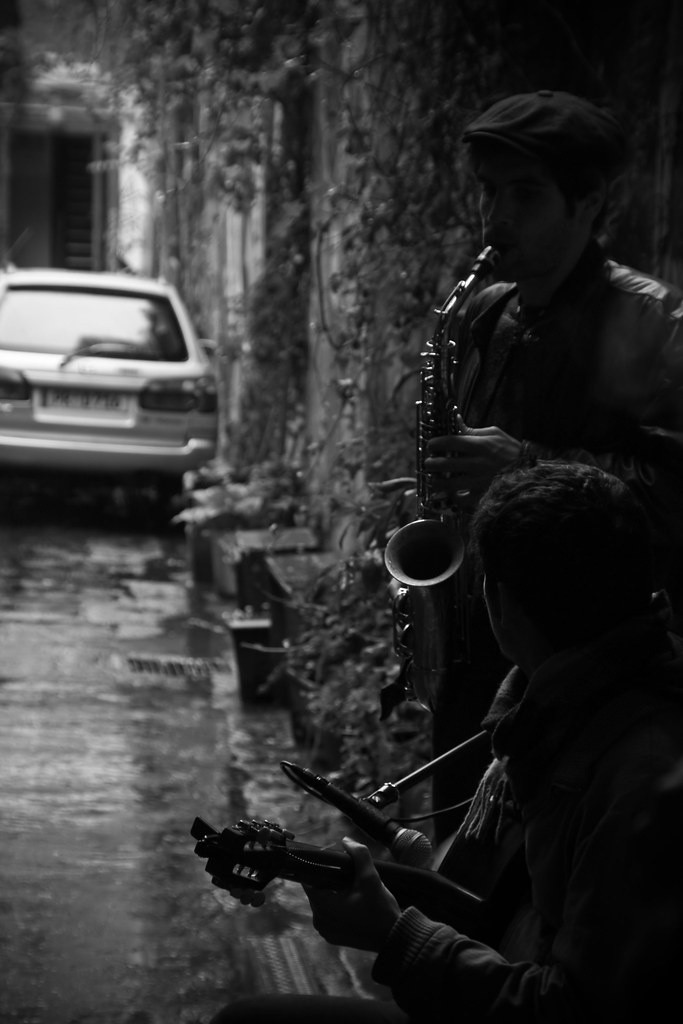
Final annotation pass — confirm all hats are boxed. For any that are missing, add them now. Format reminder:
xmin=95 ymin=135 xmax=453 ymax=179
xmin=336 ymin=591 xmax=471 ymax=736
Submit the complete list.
xmin=461 ymin=90 xmax=635 ymax=191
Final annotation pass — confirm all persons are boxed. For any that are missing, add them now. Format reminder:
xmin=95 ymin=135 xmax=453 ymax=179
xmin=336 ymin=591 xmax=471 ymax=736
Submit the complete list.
xmin=210 ymin=458 xmax=682 ymax=1023
xmin=419 ymin=82 xmax=682 ymax=857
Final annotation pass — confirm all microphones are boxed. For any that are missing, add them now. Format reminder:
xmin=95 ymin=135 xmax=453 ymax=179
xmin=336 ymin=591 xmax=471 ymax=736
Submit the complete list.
xmin=287 ymin=764 xmax=432 ymax=868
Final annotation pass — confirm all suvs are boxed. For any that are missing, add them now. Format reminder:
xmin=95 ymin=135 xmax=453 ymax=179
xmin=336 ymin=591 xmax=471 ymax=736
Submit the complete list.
xmin=0 ymin=268 xmax=221 ymax=530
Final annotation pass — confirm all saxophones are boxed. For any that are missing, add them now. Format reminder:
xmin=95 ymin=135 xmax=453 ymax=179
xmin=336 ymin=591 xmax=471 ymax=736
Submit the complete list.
xmin=382 ymin=240 xmax=503 ymax=716
